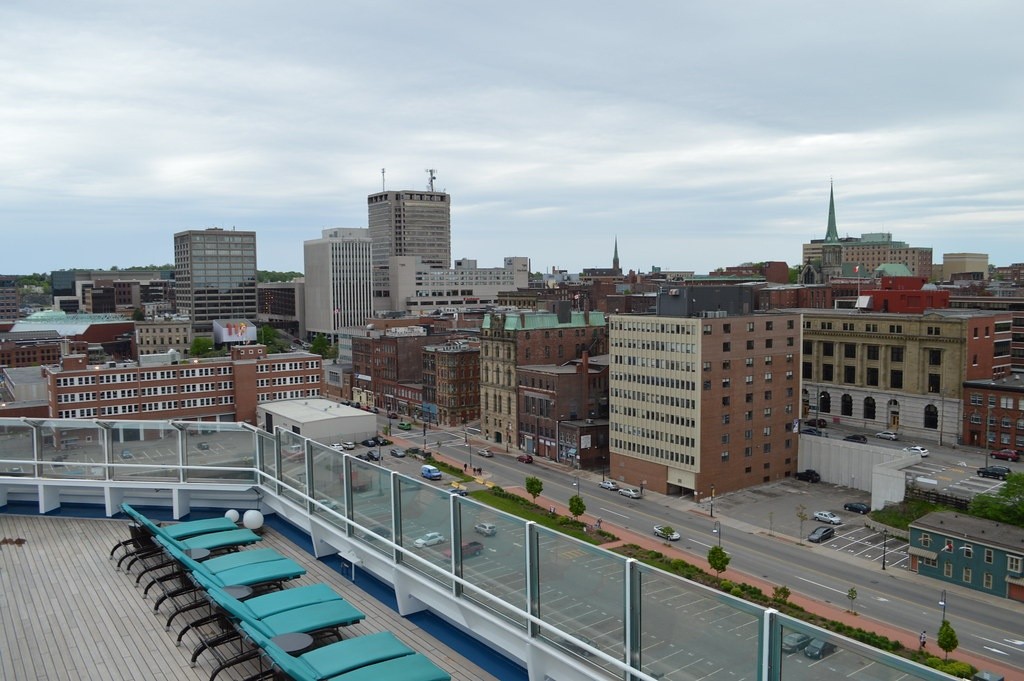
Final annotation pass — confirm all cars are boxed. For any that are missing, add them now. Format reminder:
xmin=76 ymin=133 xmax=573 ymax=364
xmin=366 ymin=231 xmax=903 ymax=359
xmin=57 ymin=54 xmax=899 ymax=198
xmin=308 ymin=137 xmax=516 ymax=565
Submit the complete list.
xmin=341 ymin=400 xmax=378 ymax=413
xmin=461 ymin=540 xmax=485 ymax=560
xmin=356 ymin=527 xmax=390 ymax=541
xmin=52 ymin=455 xmax=63 ymax=467
xmin=807 ymin=503 xmax=871 ymax=542
xmin=313 ymin=500 xmax=339 ymax=512
xmin=440 ymin=488 xmax=469 ymax=499
xmin=654 ymin=525 xmax=681 ymax=540
xmin=198 ymin=442 xmax=209 ymax=450
xmin=977 ymin=449 xmax=1020 ymax=480
xmin=329 ymin=436 xmax=405 ymax=462
xmin=414 ymin=532 xmax=445 ymax=548
xmin=122 ymin=448 xmax=131 ymax=459
xmin=477 ymin=449 xmax=493 ymax=457
xmin=517 ymin=454 xmax=533 ymax=463
xmin=598 ymin=480 xmax=642 ymax=498
xmin=800 ymin=418 xmax=829 ymax=437
xmin=387 ymin=413 xmax=398 ymax=419
xmin=842 ymin=430 xmax=930 ymax=458
xmin=782 ymin=633 xmax=838 ymax=659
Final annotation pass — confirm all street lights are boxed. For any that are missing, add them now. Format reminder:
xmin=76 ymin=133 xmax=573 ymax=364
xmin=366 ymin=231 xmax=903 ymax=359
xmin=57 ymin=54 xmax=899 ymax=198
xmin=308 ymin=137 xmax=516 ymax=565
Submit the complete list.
xmin=602 ymin=455 xmax=606 ymax=481
xmin=713 ymin=521 xmax=721 ymax=547
xmin=882 ymin=528 xmax=889 ymax=569
xmin=572 ymin=475 xmax=579 ymax=496
xmin=985 ymin=405 xmax=996 ymax=467
xmin=931 ymin=385 xmax=944 ymax=445
xmin=506 ymin=430 xmax=513 ymax=452
xmin=939 ymin=590 xmax=947 ymax=619
xmin=465 ymin=443 xmax=472 ymax=467
xmin=806 ymin=382 xmax=819 ymax=418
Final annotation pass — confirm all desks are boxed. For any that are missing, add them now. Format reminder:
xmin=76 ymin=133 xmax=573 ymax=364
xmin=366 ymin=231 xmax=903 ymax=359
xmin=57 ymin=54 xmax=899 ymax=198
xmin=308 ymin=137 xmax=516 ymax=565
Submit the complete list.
xmin=178 ymin=549 xmax=210 ymax=589
xmin=218 ymin=584 xmax=253 ymax=628
xmin=128 ymin=519 xmax=161 ymax=548
xmin=269 ymin=633 xmax=314 ymax=658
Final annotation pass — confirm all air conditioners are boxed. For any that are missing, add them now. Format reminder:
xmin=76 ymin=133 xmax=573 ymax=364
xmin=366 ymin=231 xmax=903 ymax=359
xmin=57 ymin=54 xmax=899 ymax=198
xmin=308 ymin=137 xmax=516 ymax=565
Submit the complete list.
xmin=106 ymin=360 xmax=117 ymax=369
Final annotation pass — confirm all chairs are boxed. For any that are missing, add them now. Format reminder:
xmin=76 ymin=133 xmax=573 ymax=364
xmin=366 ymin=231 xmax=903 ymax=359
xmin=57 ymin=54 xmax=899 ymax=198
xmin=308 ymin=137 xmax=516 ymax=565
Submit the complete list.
xmin=108 ymin=499 xmax=448 ymax=681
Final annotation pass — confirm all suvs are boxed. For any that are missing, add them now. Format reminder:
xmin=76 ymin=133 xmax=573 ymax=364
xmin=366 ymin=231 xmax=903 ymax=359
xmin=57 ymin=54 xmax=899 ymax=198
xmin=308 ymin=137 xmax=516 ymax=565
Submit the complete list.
xmin=475 ymin=522 xmax=497 ymax=537
xmin=397 ymin=422 xmax=411 ymax=430
xmin=795 ymin=469 xmax=820 ymax=483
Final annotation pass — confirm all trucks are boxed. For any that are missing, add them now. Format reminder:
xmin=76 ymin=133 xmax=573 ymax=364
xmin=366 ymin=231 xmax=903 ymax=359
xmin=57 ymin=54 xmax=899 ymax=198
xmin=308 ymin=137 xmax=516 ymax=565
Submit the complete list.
xmin=338 ymin=464 xmax=373 ymax=492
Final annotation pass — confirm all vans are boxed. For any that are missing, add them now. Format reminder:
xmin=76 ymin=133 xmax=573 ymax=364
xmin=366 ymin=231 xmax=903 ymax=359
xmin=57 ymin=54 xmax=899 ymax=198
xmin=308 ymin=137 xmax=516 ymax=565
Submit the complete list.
xmin=407 ymin=447 xmax=431 ymax=457
xmin=420 ymin=464 xmax=442 ymax=480
